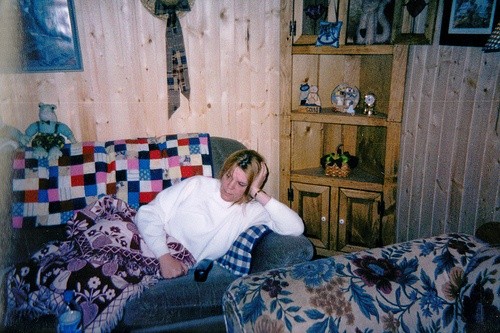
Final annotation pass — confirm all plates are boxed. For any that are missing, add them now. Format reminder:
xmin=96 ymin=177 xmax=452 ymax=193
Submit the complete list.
xmin=332 ymin=82 xmax=360 ymax=113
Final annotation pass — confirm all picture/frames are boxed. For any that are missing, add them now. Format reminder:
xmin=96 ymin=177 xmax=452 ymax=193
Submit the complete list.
xmin=17 ymin=0 xmax=84 ymax=72
xmin=439 ymin=0 xmax=500 ymax=46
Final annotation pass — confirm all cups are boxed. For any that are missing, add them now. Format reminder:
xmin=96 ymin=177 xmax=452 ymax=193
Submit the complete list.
xmin=58 ymin=311 xmax=83 ymax=333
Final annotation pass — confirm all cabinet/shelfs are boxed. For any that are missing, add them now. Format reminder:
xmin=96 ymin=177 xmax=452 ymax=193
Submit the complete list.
xmin=277 ymin=0 xmax=416 ymax=258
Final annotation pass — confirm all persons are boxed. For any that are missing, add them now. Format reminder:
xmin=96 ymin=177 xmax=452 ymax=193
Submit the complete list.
xmin=135 ymin=149 xmax=304 ymax=278
xmin=457 ymin=0 xmax=489 ymax=23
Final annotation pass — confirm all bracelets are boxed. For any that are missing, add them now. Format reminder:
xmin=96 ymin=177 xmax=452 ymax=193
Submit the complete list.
xmin=254 ymin=190 xmax=262 ymax=200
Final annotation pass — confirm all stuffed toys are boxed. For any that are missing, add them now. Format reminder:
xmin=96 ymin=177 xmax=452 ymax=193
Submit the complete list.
xmin=25 ymin=102 xmax=76 ymax=159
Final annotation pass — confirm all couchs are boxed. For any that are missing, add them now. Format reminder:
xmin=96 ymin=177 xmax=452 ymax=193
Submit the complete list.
xmin=221 ymin=231 xmax=500 ymax=333
xmin=11 ymin=133 xmax=313 ymax=333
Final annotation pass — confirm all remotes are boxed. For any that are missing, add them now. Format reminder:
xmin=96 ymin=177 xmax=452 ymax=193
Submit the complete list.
xmin=194 ymin=259 xmax=213 ymax=281
